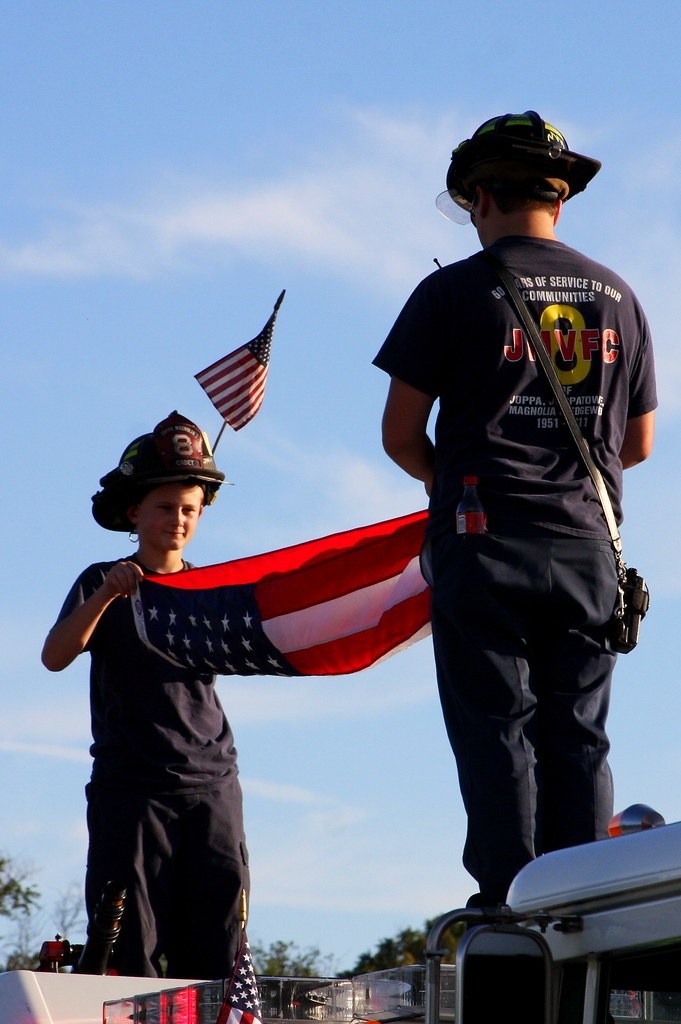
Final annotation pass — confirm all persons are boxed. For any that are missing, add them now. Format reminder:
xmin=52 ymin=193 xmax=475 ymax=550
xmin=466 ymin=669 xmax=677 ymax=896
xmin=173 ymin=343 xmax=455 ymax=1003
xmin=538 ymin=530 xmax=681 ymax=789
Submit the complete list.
xmin=41 ymin=409 xmax=252 ymax=979
xmin=370 ymin=110 xmax=659 ymax=928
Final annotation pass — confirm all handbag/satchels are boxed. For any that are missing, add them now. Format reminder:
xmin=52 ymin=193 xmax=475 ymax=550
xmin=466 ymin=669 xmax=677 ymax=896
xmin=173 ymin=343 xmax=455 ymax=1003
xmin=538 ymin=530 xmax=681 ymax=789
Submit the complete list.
xmin=611 ymin=568 xmax=650 ymax=654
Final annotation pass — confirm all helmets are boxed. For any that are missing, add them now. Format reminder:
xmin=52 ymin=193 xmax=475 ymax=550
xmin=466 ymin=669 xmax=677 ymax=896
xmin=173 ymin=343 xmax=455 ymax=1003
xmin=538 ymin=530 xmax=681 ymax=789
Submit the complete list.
xmin=92 ymin=410 xmax=227 ymax=532
xmin=445 ymin=107 xmax=602 ymax=215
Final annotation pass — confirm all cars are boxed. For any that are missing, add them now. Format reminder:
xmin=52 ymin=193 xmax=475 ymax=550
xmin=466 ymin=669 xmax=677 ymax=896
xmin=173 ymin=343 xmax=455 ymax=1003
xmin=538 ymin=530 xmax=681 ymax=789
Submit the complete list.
xmin=1 ymin=941 xmax=350 ymax=1023
xmin=352 ymin=801 xmax=681 ymax=1024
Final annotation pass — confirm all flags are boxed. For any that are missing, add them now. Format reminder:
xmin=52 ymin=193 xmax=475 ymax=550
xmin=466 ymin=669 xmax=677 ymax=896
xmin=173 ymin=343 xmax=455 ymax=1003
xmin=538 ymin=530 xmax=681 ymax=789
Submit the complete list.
xmin=129 ymin=504 xmax=431 ymax=678
xmin=193 ymin=310 xmax=278 ymax=432
xmin=216 ymin=927 xmax=262 ymax=1024
